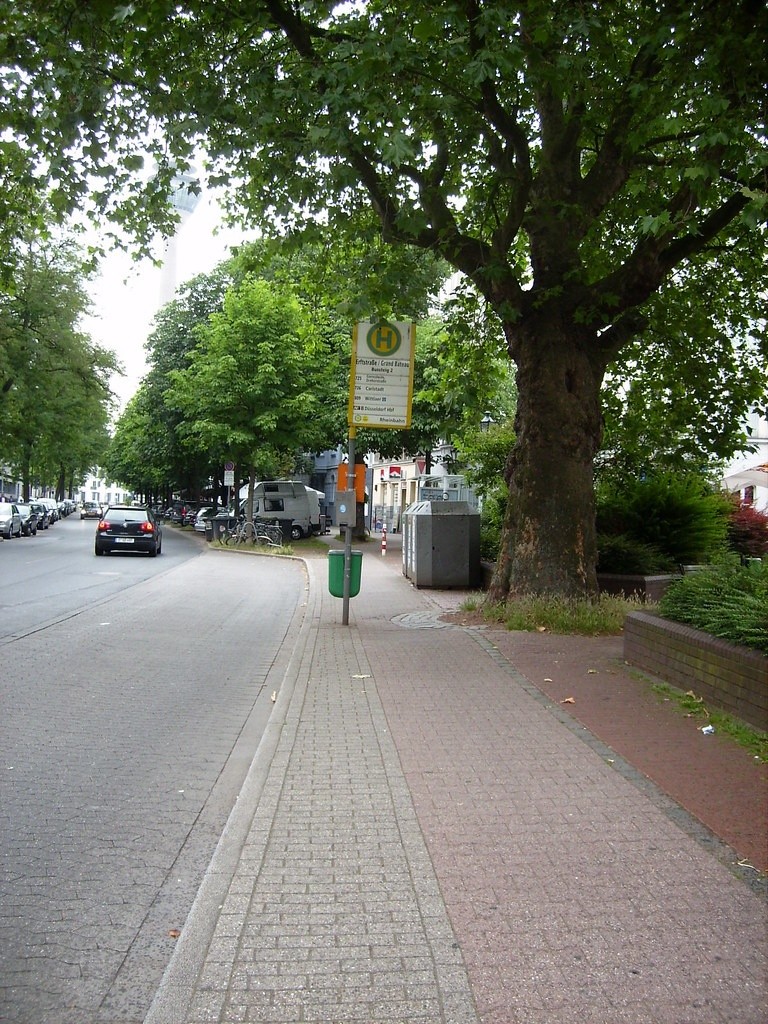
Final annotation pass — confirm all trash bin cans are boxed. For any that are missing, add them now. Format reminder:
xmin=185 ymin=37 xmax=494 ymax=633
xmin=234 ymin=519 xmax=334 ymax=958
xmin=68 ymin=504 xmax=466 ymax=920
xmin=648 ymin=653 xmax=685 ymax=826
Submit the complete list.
xmin=223 ymin=516 xmax=237 ymax=530
xmin=210 ymin=516 xmax=228 ymax=539
xmin=327 ymin=548 xmax=363 ymax=598
xmin=401 ymin=500 xmax=481 ymax=590
xmin=202 ymin=518 xmax=213 ymax=542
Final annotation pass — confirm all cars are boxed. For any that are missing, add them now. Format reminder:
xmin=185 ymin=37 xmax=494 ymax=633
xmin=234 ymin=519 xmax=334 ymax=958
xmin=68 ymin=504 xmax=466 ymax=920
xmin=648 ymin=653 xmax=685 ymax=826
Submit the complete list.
xmin=0 ymin=502 xmax=23 ymax=540
xmin=34 ymin=497 xmax=83 ymax=525
xmin=133 ymin=477 xmax=332 ymax=547
xmin=14 ymin=502 xmax=38 ymax=536
xmin=95 ymin=504 xmax=163 ymax=557
xmin=79 ymin=500 xmax=104 ymax=520
xmin=28 ymin=502 xmax=50 ymax=531
